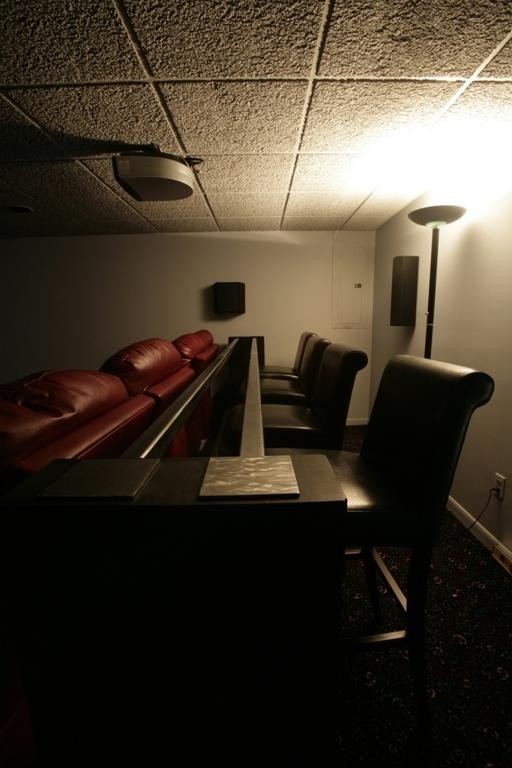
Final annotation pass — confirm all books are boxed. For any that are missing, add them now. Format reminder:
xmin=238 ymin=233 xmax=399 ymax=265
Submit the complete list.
xmin=41 ymin=453 xmax=163 ymax=501
xmin=198 ymin=454 xmax=300 ymax=501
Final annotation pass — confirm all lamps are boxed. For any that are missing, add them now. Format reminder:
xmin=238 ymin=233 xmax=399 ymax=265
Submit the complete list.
xmin=406 ymin=203 xmax=467 ymax=361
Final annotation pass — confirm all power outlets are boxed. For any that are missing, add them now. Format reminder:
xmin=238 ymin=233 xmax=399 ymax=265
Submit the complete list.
xmin=492 ymin=471 xmax=506 ymax=500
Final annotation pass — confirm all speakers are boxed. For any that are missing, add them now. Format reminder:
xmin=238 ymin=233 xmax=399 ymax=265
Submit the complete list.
xmin=213 ymin=281 xmax=247 ymax=317
xmin=389 ymin=254 xmax=418 ymax=328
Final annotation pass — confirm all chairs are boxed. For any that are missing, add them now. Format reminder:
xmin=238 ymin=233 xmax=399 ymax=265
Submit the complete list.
xmin=2 ymin=330 xmax=223 ymax=483
xmin=264 ymin=355 xmax=493 ymax=706
xmin=256 ymin=331 xmax=370 ymax=446
